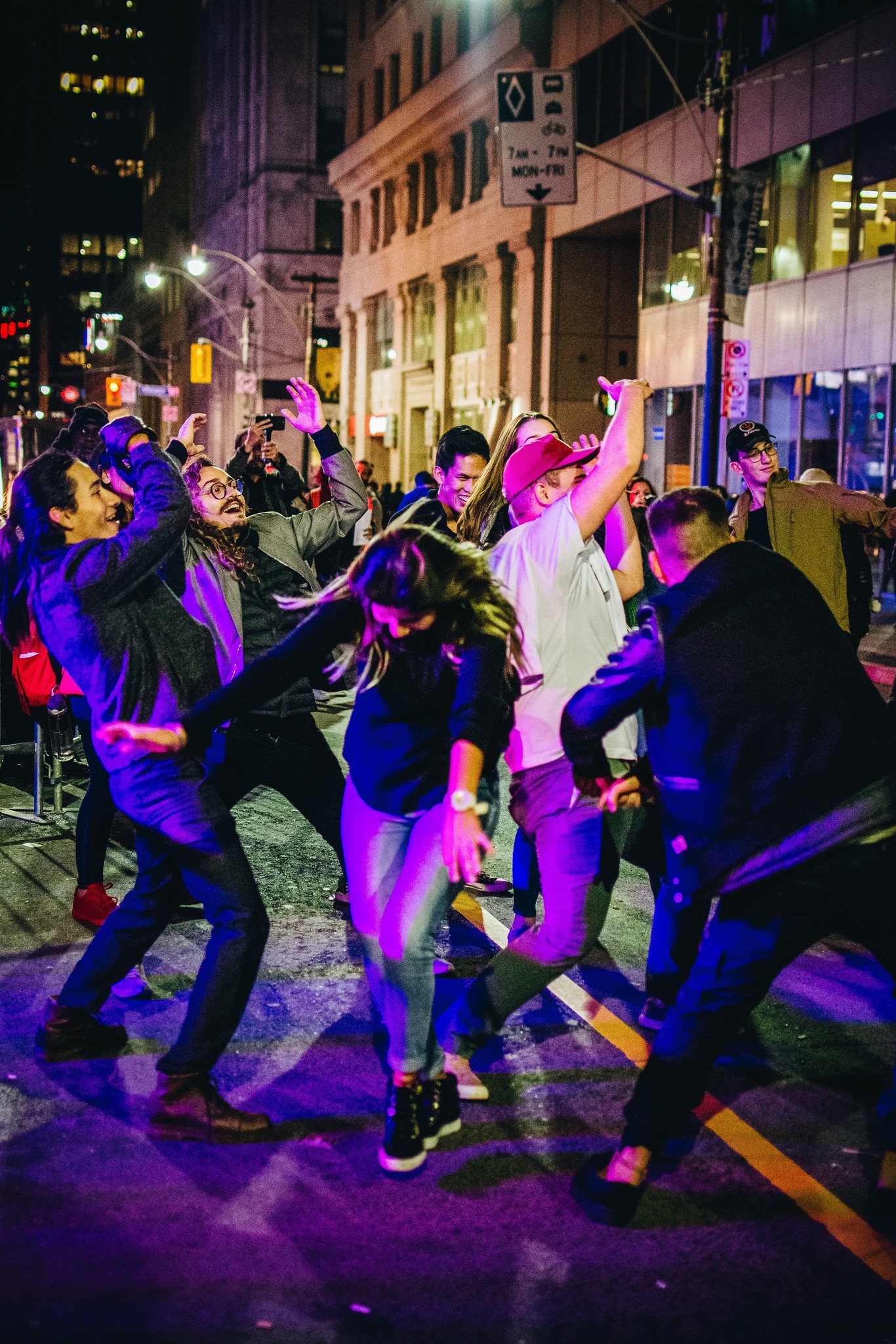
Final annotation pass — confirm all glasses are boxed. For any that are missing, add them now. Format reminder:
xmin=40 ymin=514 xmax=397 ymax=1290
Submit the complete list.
xmin=742 ymin=441 xmax=779 ymax=463
xmin=199 ymin=478 xmax=244 ymax=498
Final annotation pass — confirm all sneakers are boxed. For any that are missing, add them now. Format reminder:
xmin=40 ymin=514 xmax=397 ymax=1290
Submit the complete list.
xmin=145 ymin=1065 xmax=271 ymax=1145
xmin=37 ymin=1010 xmax=130 ymax=1054
xmin=574 ymin=1155 xmax=647 ymax=1226
xmin=466 ymin=868 xmax=512 ymax=894
xmin=70 ymin=882 xmax=118 ymax=929
xmin=443 ymin=1050 xmax=489 ymax=1105
xmin=379 ymin=1083 xmax=426 ymax=1169
xmin=417 ymin=1070 xmax=464 ymax=1148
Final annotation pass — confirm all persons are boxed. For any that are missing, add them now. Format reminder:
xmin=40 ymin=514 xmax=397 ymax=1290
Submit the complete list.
xmin=561 ymin=483 xmax=896 ymax=1225
xmin=287 ymin=442 xmax=876 ymax=659
xmin=0 ymin=416 xmax=274 ymax=1147
xmin=91 ymin=526 xmax=527 ymax=1172
xmin=9 ymin=441 xmax=188 ymax=926
xmin=54 ymin=399 xmax=113 ymax=485
xmin=386 ymin=425 xmax=515 ymax=896
xmin=108 ymin=376 xmax=371 ymax=1002
xmin=456 ymin=411 xmax=562 ymax=895
xmin=726 ymin=421 xmax=896 ymax=648
xmin=224 ymin=418 xmax=305 ymax=522
xmin=437 ymin=375 xmax=658 ymax=1101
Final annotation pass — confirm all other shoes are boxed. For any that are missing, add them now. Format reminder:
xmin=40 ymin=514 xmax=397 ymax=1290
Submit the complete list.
xmin=335 ymin=874 xmax=354 ymax=902
xmin=638 ymin=998 xmax=670 ymax=1032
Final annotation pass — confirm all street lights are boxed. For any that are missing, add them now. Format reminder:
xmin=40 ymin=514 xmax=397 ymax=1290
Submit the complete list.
xmin=58 ymin=246 xmax=319 ymax=480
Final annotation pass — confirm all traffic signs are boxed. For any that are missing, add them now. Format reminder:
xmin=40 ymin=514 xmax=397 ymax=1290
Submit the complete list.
xmin=493 ymin=69 xmax=580 ymax=207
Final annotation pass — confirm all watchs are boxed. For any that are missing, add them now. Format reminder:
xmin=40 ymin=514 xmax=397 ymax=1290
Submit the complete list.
xmin=441 ymin=788 xmax=489 ymax=818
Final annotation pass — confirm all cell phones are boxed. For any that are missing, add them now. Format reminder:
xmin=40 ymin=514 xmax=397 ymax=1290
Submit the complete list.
xmin=255 ymin=415 xmax=285 ymax=430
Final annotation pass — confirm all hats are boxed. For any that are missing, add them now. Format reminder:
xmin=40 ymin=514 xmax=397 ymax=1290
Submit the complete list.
xmin=500 ymin=431 xmax=602 ymax=501
xmin=724 ymin=420 xmax=777 ymax=456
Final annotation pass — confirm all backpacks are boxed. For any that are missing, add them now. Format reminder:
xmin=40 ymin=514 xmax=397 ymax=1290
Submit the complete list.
xmin=11 ymin=620 xmax=71 ymax=715
